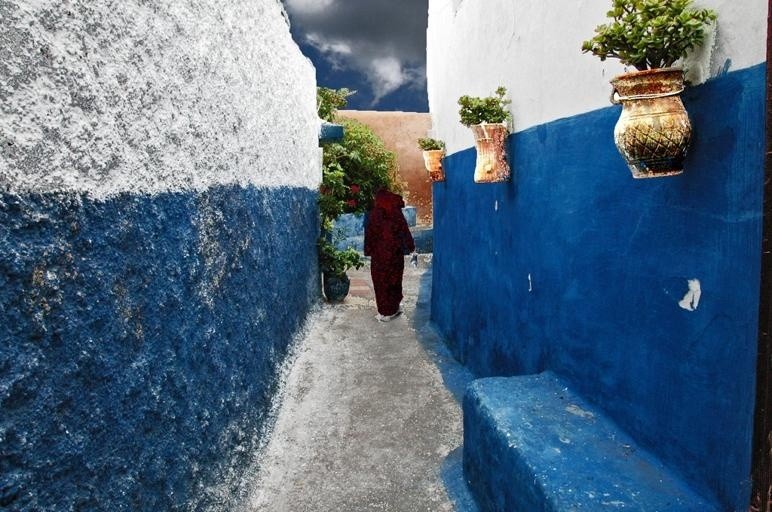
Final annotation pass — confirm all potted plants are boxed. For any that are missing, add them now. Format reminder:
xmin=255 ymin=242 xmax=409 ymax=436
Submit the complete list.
xmin=582 ymin=0 xmax=718 ymax=179
xmin=318 ymin=238 xmax=364 ymax=303
xmin=458 ymin=85 xmax=512 ymax=184
xmin=417 ymin=137 xmax=447 ymax=182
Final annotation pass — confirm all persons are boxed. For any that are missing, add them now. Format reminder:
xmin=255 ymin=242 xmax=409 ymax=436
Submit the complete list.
xmin=362 ymin=187 xmax=416 ymax=322
